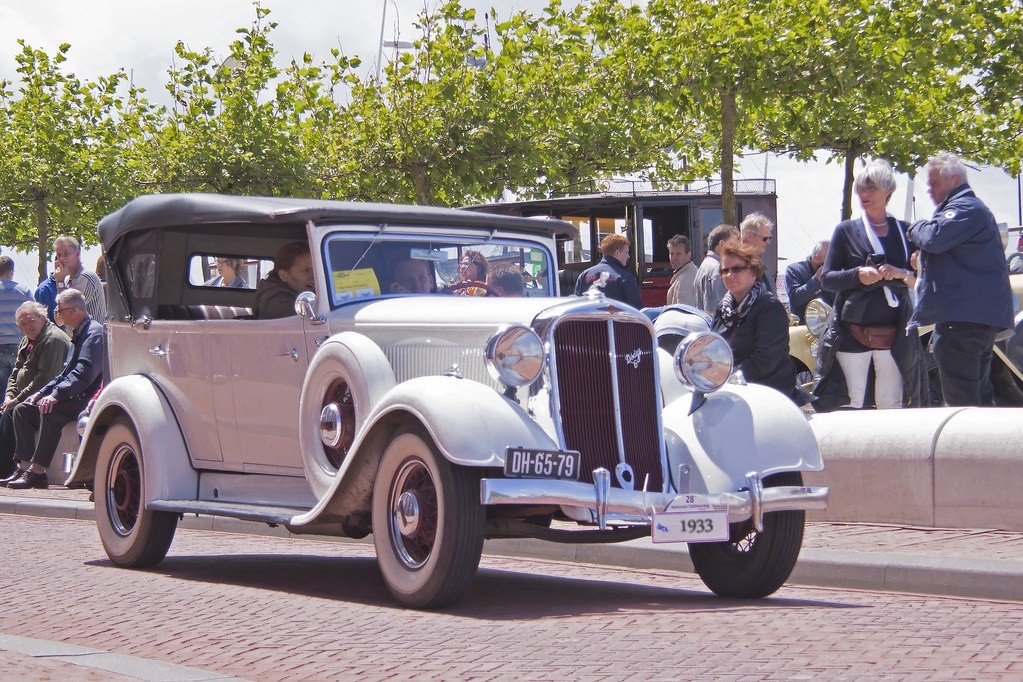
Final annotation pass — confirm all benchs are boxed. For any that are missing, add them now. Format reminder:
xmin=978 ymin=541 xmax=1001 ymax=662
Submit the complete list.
xmin=155 ymin=305 xmax=254 ymax=321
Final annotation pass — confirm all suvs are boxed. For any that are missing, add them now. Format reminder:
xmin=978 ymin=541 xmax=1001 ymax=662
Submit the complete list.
xmin=52 ymin=195 xmax=831 ymax=608
xmin=452 ymin=178 xmax=781 ymax=330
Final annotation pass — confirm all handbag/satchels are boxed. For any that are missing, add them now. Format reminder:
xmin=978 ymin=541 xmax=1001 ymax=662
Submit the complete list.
xmin=845 ymin=321 xmax=898 ymax=350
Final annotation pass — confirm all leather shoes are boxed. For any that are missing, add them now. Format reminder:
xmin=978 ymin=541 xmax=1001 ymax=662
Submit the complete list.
xmin=6 ymin=470 xmax=50 ymax=489
xmin=0 ymin=469 xmax=25 ymax=487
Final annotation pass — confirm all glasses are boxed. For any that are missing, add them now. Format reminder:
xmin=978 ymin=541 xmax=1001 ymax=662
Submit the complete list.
xmin=58 ymin=308 xmax=70 ymax=313
xmin=217 ymin=260 xmax=228 ymax=265
xmin=755 ymin=235 xmax=771 ymax=242
xmin=719 ymin=265 xmax=752 ymax=275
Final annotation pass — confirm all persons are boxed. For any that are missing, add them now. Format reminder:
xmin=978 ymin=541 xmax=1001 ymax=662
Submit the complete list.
xmin=708 ymin=240 xmax=818 ymax=408
xmin=784 ymin=241 xmax=834 ymax=326
xmin=807 ymin=162 xmax=929 ymax=410
xmin=574 ymin=234 xmax=643 ymax=311
xmin=251 ymin=241 xmax=316 ymax=320
xmin=454 ymin=249 xmax=524 ymax=297
xmin=210 ymin=257 xmax=250 ymax=288
xmin=385 ymin=246 xmax=434 ymax=293
xmin=666 ymin=234 xmax=699 ymax=306
xmin=0 ymin=238 xmax=109 ymax=489
xmin=740 ymin=211 xmax=777 ymax=297
xmin=907 ymin=153 xmax=1013 ymax=410
xmin=693 ymin=223 xmax=741 ymax=312
